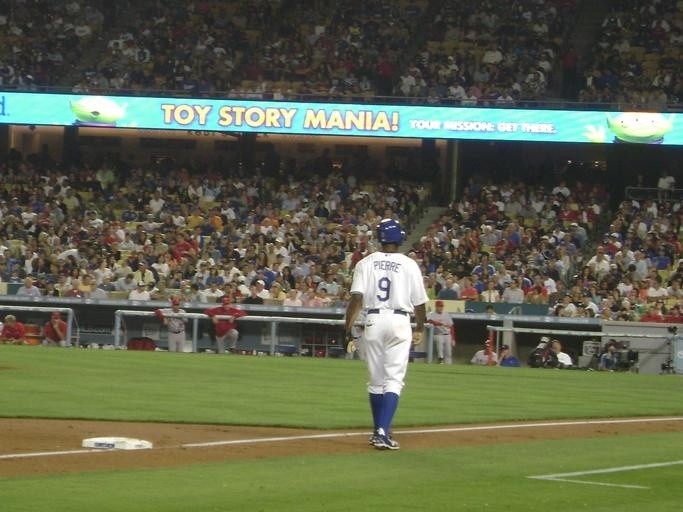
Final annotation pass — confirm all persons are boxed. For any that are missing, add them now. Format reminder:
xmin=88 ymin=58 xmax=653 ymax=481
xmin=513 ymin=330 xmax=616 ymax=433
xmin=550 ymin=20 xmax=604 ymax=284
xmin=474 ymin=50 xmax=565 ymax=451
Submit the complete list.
xmin=342 ymin=219 xmax=430 ymax=449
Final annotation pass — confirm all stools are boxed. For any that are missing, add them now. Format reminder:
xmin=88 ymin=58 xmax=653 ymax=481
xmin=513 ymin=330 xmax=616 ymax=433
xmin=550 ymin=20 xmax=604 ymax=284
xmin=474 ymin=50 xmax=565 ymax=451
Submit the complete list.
xmin=368 ymin=309 xmax=406 ymax=315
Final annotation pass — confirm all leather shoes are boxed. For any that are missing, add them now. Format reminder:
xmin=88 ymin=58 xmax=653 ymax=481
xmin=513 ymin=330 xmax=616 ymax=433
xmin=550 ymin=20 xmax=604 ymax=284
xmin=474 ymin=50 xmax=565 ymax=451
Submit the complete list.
xmin=344 ymin=328 xmax=357 ymax=353
xmin=412 ymin=332 xmax=424 ymax=345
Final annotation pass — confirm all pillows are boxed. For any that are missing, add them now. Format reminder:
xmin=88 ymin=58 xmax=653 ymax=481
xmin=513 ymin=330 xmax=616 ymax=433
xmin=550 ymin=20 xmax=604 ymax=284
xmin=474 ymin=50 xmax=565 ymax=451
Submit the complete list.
xmin=377 ymin=218 xmax=407 ymax=243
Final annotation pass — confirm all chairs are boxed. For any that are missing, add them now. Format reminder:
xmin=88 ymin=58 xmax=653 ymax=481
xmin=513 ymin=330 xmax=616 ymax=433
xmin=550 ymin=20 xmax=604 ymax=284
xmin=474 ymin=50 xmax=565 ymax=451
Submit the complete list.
xmin=368 ymin=427 xmax=400 ymax=450
